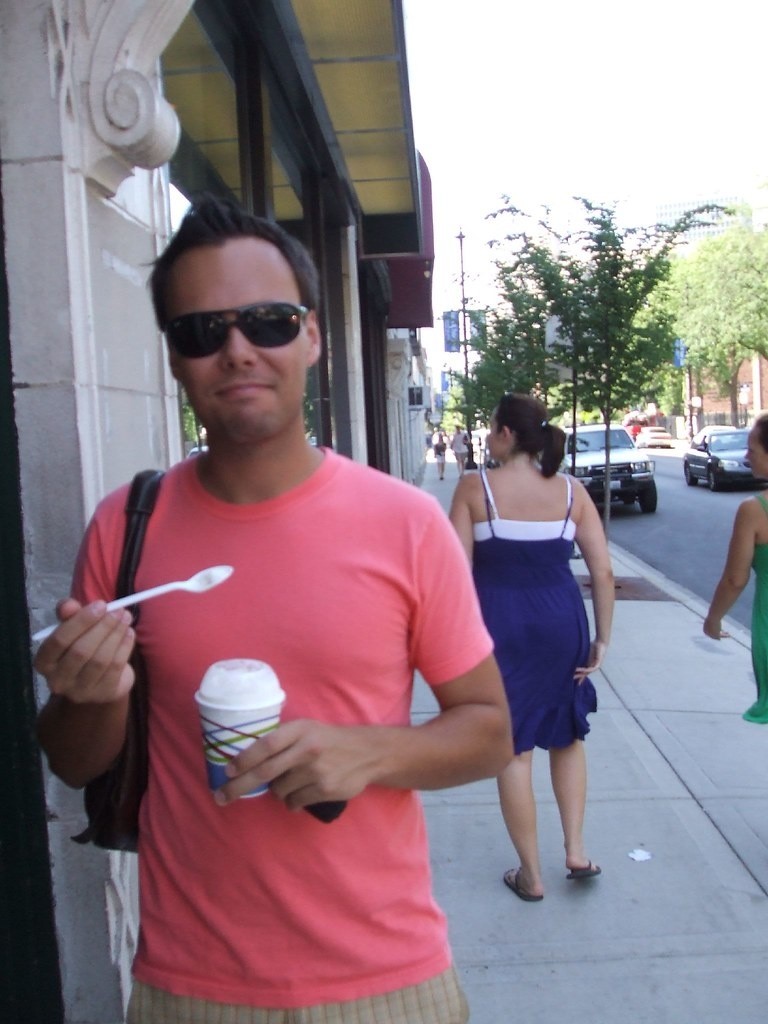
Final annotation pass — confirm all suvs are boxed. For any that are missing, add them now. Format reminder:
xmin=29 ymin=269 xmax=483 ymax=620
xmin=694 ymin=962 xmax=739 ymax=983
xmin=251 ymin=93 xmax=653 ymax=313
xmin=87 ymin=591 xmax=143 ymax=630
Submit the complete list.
xmin=556 ymin=423 xmax=657 ymax=514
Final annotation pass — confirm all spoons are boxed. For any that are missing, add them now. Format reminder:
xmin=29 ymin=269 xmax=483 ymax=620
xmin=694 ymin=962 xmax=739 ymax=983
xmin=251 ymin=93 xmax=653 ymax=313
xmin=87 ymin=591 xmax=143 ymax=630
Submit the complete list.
xmin=32 ymin=565 xmax=237 ymax=644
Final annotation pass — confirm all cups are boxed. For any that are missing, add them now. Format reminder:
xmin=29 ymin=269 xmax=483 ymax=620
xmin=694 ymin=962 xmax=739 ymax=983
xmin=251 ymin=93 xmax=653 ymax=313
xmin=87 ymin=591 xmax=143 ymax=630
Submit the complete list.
xmin=194 ymin=658 xmax=287 ymax=800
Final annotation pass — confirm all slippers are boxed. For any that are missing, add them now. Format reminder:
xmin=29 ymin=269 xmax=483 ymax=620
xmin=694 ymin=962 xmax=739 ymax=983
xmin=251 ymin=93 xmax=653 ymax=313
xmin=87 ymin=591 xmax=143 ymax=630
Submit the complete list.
xmin=503 ymin=868 xmax=543 ymax=901
xmin=566 ymin=860 xmax=601 ymax=879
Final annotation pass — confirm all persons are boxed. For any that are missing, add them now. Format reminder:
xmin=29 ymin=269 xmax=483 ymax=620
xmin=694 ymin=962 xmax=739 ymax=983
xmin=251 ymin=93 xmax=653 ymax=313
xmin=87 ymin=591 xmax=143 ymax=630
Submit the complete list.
xmin=703 ymin=413 xmax=768 ymax=724
xmin=632 ymin=422 xmax=641 ymax=442
xmin=36 ymin=208 xmax=515 ymax=1024
xmin=451 ymin=426 xmax=470 ymax=478
xmin=449 ymin=394 xmax=615 ymax=901
xmin=432 ymin=431 xmax=449 ymax=480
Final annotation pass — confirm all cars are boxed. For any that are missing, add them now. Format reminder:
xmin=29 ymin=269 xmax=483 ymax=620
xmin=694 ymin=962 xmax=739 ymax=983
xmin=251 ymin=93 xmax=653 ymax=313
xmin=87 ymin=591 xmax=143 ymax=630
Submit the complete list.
xmin=682 ymin=427 xmax=768 ymax=492
xmin=632 ymin=427 xmax=671 ymax=449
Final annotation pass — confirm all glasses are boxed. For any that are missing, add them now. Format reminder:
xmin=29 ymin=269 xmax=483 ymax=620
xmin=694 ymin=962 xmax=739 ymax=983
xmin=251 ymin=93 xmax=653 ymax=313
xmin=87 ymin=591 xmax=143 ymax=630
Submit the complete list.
xmin=164 ymin=302 xmax=309 ymax=357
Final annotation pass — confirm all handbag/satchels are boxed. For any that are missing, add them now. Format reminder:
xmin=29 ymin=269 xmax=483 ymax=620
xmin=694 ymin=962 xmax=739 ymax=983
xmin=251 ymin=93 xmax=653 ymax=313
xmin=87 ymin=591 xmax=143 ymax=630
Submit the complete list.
xmin=71 ymin=470 xmax=163 ymax=853
